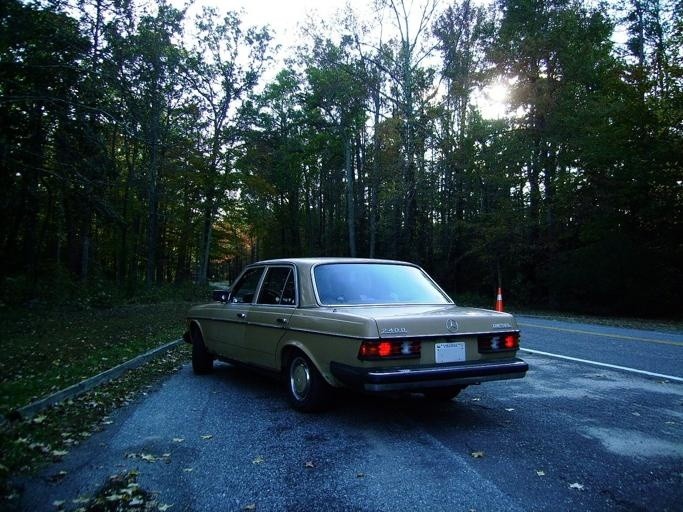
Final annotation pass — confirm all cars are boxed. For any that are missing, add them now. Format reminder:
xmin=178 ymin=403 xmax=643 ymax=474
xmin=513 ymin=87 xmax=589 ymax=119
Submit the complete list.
xmin=183 ymin=257 xmax=530 ymax=411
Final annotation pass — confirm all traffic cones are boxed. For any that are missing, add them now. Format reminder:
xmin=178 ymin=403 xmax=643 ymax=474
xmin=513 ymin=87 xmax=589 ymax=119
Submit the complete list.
xmin=492 ymin=287 xmax=506 ymax=313
